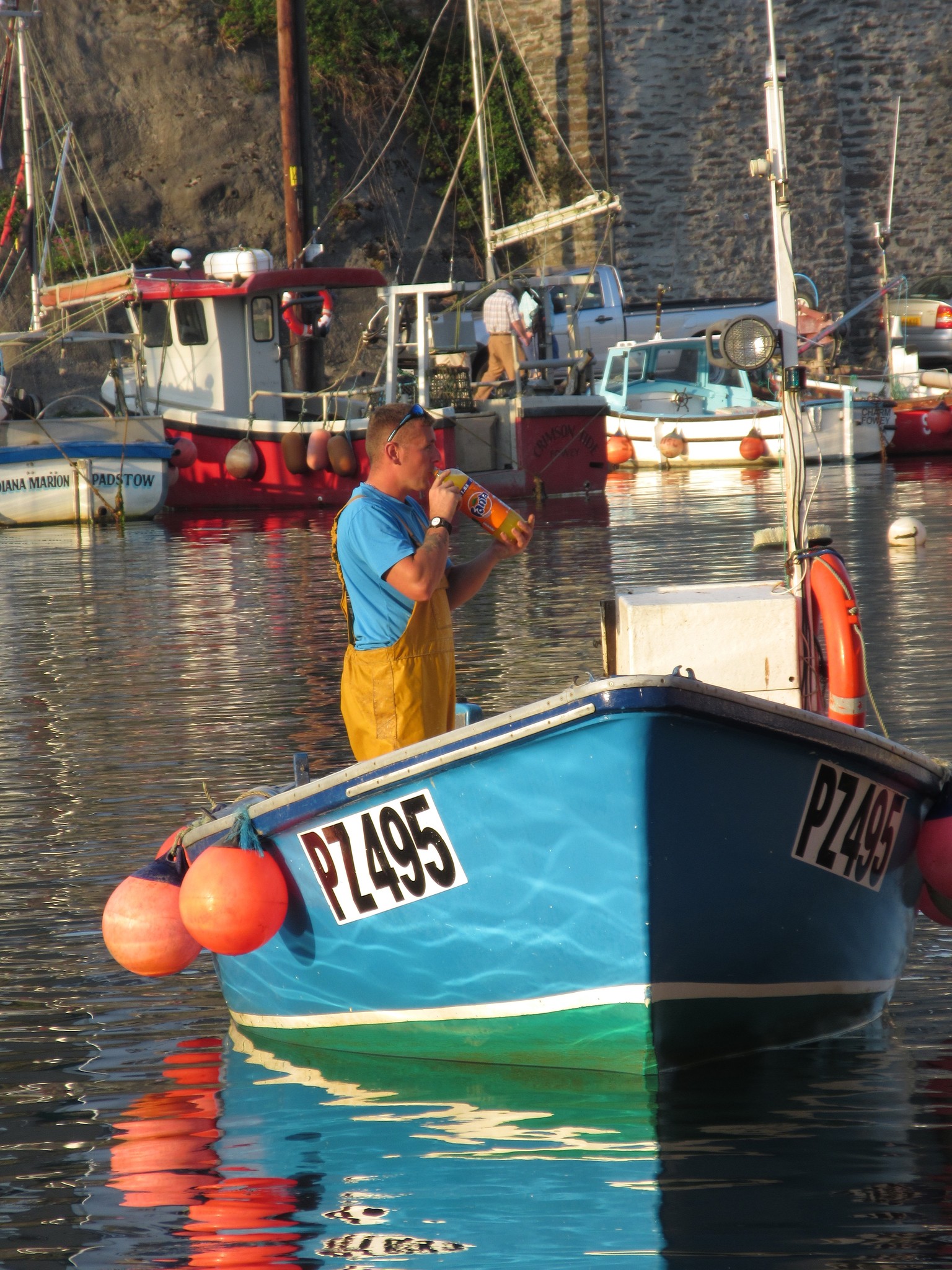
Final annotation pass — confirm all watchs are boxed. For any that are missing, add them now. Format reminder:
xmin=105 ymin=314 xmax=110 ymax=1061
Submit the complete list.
xmin=427 ymin=516 xmax=452 ymax=535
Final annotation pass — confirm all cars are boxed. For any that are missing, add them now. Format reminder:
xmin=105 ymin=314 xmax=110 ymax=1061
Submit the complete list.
xmin=875 ymin=271 xmax=952 ymax=358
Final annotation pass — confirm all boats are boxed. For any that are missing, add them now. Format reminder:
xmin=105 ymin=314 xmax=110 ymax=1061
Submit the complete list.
xmin=0 ymin=329 xmax=183 ymax=524
xmin=759 ymin=305 xmax=952 ymax=451
xmin=38 ymin=247 xmax=615 ymax=513
xmin=593 ymin=328 xmax=898 ymax=473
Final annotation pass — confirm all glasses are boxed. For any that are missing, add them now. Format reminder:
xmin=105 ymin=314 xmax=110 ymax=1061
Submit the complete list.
xmin=387 ymin=404 xmax=423 ymax=441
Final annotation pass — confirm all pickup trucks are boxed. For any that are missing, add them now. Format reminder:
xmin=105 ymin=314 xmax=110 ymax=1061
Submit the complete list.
xmin=397 ymin=265 xmax=782 ymax=388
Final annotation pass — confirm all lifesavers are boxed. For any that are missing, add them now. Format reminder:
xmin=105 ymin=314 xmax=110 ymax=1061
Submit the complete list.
xmin=281 ymin=289 xmax=333 ymax=337
xmin=802 ymin=546 xmax=865 ymax=730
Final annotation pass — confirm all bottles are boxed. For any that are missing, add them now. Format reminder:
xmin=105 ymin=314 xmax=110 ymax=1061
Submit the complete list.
xmin=432 ymin=467 xmax=526 ymax=544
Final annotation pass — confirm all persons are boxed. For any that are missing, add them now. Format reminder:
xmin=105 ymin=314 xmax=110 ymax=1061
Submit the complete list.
xmin=474 ymin=280 xmax=532 ymax=399
xmin=329 ymin=402 xmax=534 ymax=763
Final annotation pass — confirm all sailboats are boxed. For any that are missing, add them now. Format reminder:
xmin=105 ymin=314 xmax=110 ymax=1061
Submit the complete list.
xmin=106 ymin=1 xmax=951 ymax=1083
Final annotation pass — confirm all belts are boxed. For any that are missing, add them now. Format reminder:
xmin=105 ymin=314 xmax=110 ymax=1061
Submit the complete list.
xmin=490 ymin=333 xmax=511 ymax=336
